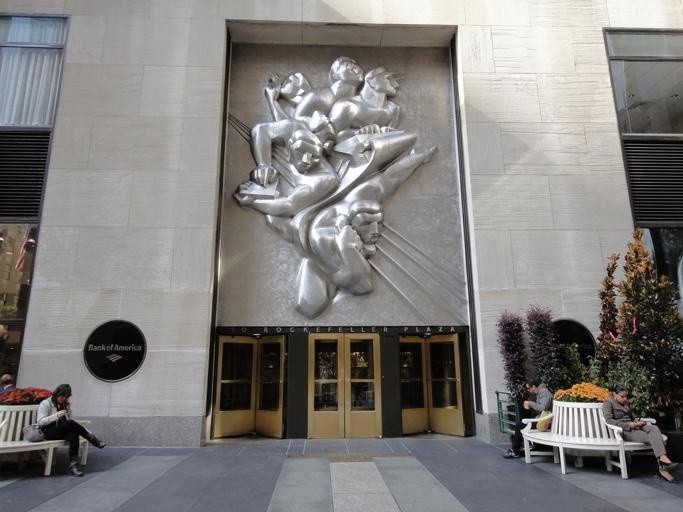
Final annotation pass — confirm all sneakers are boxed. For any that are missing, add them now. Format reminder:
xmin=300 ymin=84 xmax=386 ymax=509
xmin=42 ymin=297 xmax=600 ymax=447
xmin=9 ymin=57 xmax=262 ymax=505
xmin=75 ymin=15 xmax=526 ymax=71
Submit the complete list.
xmin=500 ymin=448 xmax=521 ymax=458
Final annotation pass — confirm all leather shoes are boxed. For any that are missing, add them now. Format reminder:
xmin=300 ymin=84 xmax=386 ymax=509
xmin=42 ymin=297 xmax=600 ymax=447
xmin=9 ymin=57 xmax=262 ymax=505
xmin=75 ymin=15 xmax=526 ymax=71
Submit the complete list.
xmin=95 ymin=440 xmax=107 ymax=448
xmin=69 ymin=462 xmax=84 ymax=476
xmin=658 ymin=458 xmax=680 ymax=471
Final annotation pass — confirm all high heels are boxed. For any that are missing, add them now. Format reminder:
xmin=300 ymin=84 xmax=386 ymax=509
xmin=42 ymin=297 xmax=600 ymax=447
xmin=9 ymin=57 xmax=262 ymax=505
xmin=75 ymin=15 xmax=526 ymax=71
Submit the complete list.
xmin=656 ymin=466 xmax=682 ymax=485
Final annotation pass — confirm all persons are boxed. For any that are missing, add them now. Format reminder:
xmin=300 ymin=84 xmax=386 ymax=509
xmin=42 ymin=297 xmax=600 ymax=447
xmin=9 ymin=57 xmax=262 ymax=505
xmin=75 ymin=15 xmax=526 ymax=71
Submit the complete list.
xmin=37 ymin=382 xmax=106 ymax=477
xmin=601 ymin=385 xmax=679 ymax=487
xmin=1 ymin=372 xmax=16 ymax=388
xmin=502 ymin=375 xmax=553 ymax=460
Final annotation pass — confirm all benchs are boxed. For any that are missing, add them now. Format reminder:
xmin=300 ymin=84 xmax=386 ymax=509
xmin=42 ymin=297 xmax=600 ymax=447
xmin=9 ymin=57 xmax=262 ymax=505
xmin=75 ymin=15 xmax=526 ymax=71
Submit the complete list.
xmin=0 ymin=403 xmax=92 ymax=476
xmin=520 ymin=397 xmax=668 ymax=480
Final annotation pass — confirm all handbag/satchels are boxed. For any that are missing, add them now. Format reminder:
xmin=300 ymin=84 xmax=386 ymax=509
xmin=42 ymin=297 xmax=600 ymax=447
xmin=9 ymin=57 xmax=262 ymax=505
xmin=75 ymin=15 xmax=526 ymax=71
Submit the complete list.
xmin=22 ymin=423 xmax=45 ymax=442
xmin=536 ymin=410 xmax=553 ymax=432
